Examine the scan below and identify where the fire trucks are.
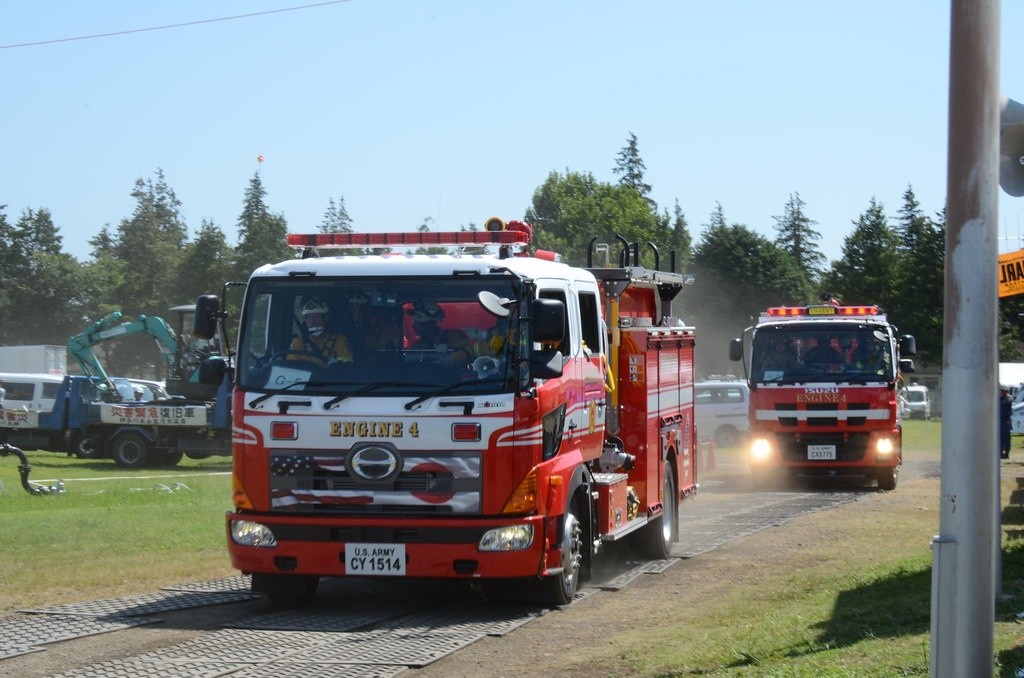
[729,299,916,493]
[193,215,701,615]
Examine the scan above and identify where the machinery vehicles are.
[65,303,233,471]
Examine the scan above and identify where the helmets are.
[345,284,380,304]
[301,299,329,315]
[840,336,851,348]
[407,299,444,323]
[774,336,788,344]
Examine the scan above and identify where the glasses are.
[413,300,424,312]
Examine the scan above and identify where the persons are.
[803,335,840,364]
[999,388,1013,458]
[286,296,354,367]
[762,334,799,371]
[348,285,401,361]
[447,327,503,364]
[855,335,890,374]
[404,300,468,354]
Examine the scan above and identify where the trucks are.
[0,345,68,375]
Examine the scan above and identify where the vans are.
[902,382,930,420]
[0,373,183,451]
[693,373,751,451]
